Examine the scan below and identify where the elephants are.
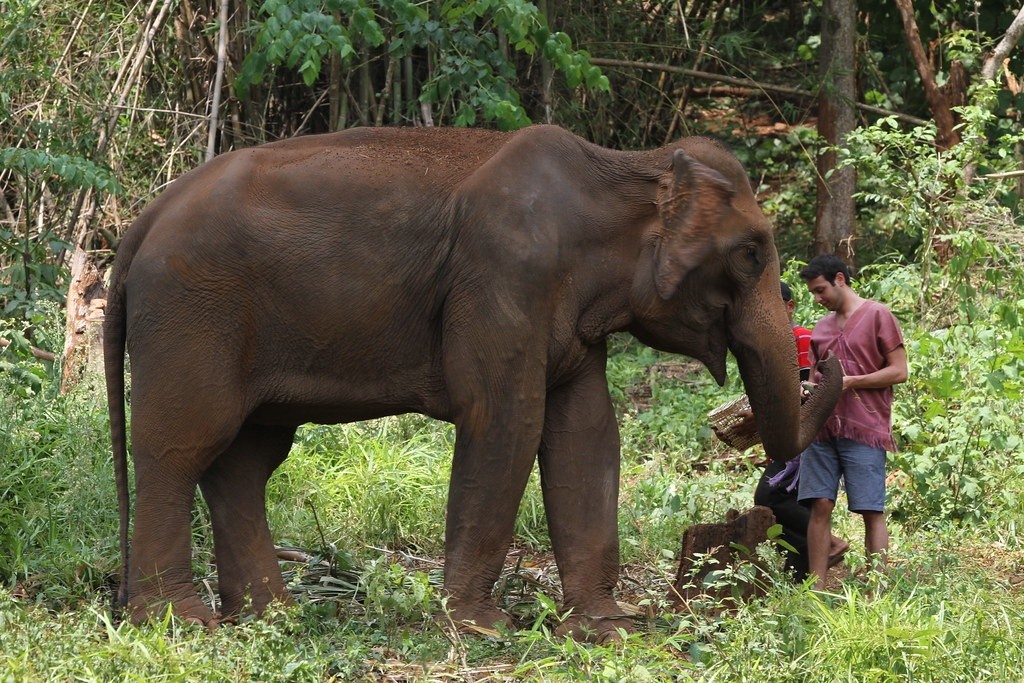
[104,136,844,648]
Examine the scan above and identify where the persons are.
[800,254,908,600]
[733,282,849,584]
